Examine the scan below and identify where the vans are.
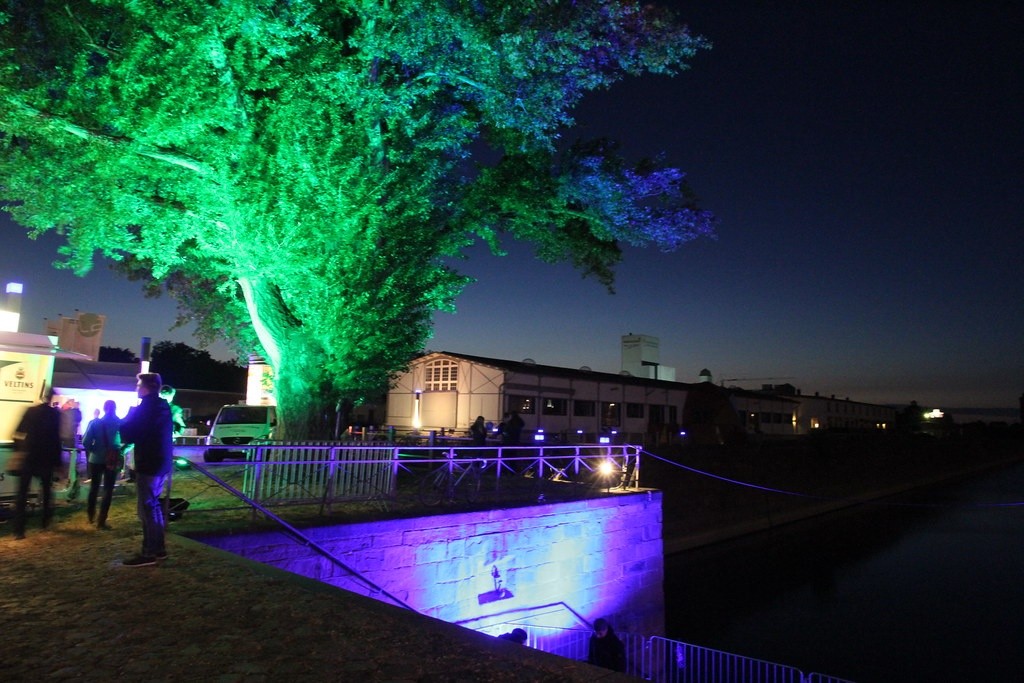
[203,405,279,462]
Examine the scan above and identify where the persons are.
[160,384,185,442]
[52,398,82,448]
[120,373,173,567]
[498,628,527,645]
[13,388,60,541]
[492,411,525,475]
[83,400,120,529]
[84,409,100,459]
[588,618,626,672]
[471,416,488,468]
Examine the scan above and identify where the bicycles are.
[419,452,486,507]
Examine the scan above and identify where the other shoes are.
[155,553,167,560]
[89,519,94,523]
[96,524,112,531]
[15,529,25,539]
[41,518,50,527]
[122,555,155,567]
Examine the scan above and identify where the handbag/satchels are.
[5,452,29,475]
[105,447,124,469]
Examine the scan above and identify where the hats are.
[43,386,58,396]
